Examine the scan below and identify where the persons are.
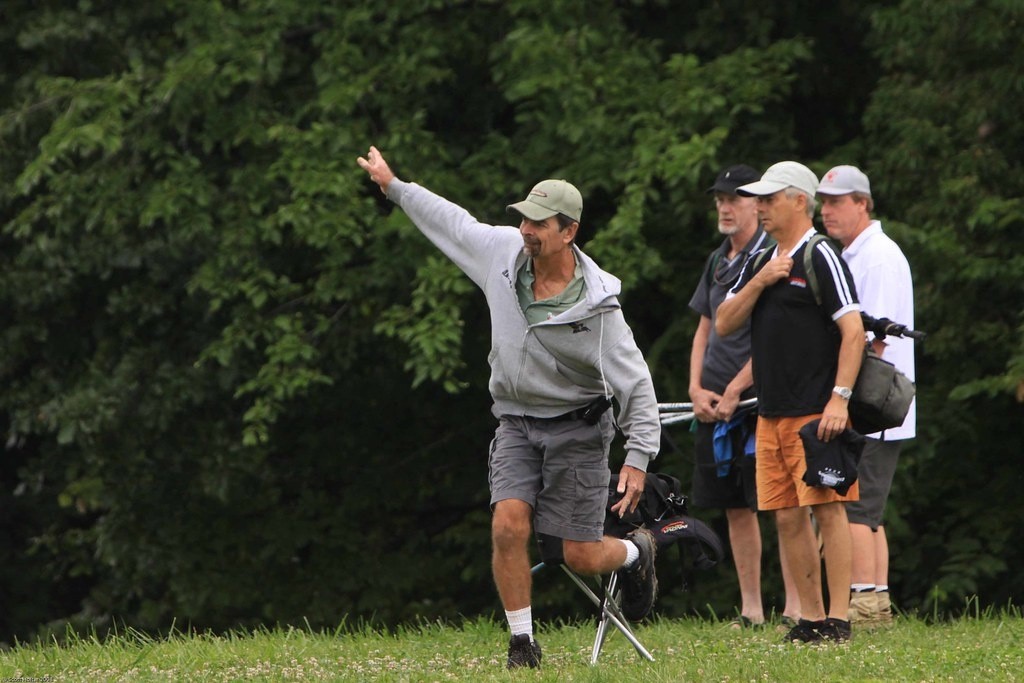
[357,144,661,672]
[814,163,917,631]
[715,160,866,644]
[688,164,802,636]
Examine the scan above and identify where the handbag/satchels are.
[847,352,915,436]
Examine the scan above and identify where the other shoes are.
[848,591,880,627]
[730,616,765,634]
[780,616,797,629]
[878,593,894,627]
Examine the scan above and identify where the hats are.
[736,160,819,197]
[506,180,583,223]
[704,164,762,195]
[817,164,871,195]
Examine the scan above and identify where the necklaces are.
[713,253,748,286]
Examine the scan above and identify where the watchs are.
[832,385,852,399]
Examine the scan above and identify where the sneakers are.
[620,529,658,621]
[812,617,852,647]
[782,618,828,644]
[506,633,542,670]
